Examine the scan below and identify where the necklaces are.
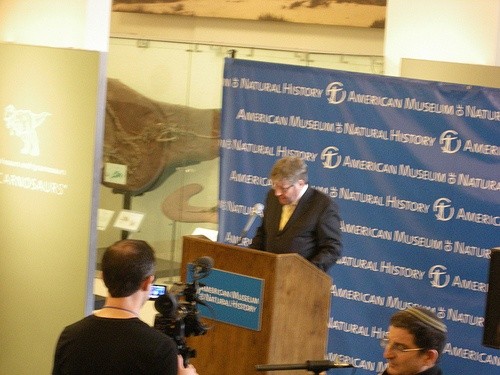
[102,305,139,316]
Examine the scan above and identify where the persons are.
[380,306,448,375]
[246,156,344,271]
[51,239,198,375]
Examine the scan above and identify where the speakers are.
[481,247,500,351]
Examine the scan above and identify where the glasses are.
[276,184,293,192]
[383,332,439,352]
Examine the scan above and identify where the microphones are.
[195,257,213,273]
[238,203,264,245]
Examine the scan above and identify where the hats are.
[404,303,448,333]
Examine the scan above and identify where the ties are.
[278,203,297,232]
